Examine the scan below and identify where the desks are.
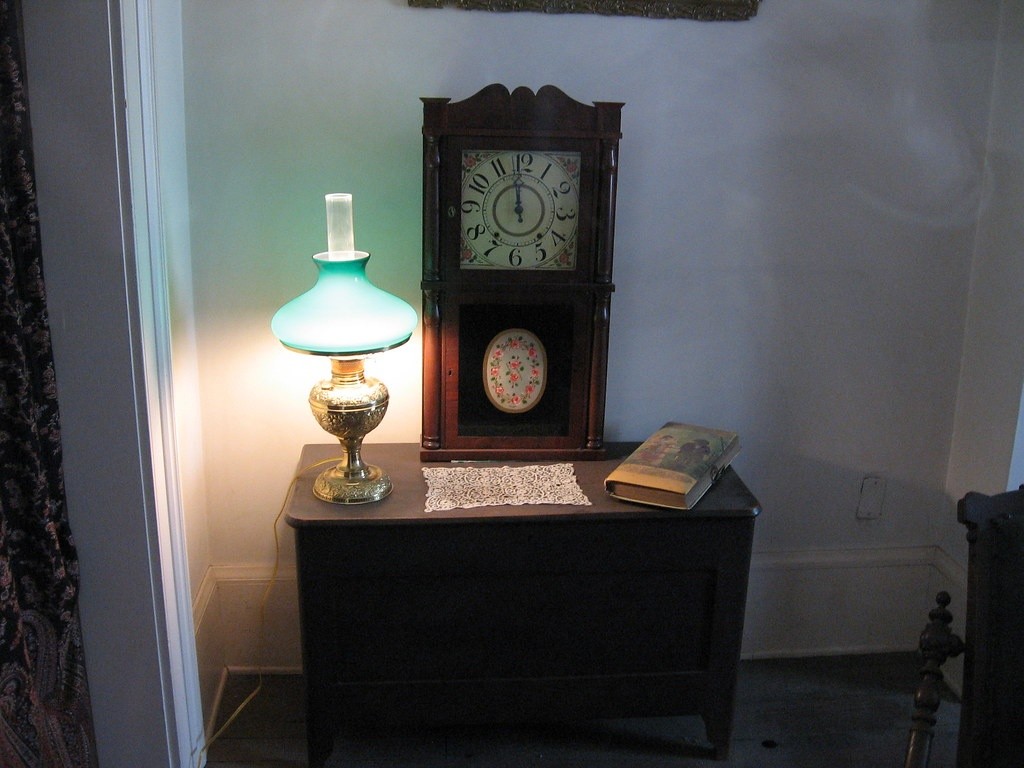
[284,443,760,768]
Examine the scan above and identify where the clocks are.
[419,84,626,462]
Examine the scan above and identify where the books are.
[602,420,743,510]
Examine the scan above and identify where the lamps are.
[272,193,418,505]
